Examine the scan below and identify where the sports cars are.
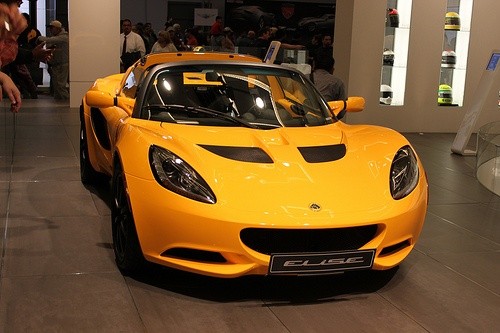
[78,51,430,281]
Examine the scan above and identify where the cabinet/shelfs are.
[438,29,470,108]
[380,23,409,106]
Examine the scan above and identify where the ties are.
[122,36,126,54]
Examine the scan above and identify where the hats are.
[167,27,175,31]
[49,20,62,27]
[224,27,234,32]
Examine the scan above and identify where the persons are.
[0,0,337,113]
[310,52,347,117]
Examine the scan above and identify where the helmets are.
[385,7,399,28]
[445,11,460,30]
[438,84,452,103]
[382,49,394,66]
[441,50,456,68]
[379,84,394,104]
